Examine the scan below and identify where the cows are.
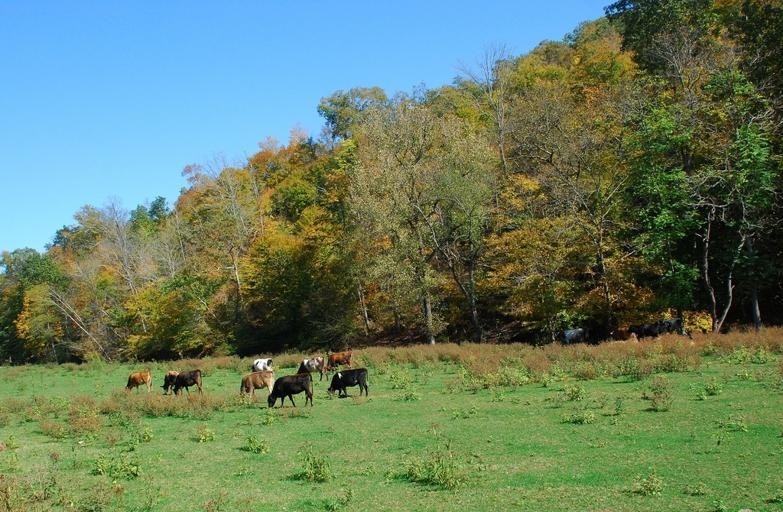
[558,326,593,347]
[160,369,203,398]
[125,371,152,392]
[240,371,273,404]
[627,318,683,338]
[297,357,328,381]
[252,358,274,372]
[326,368,368,399]
[268,373,313,407]
[324,351,352,373]
